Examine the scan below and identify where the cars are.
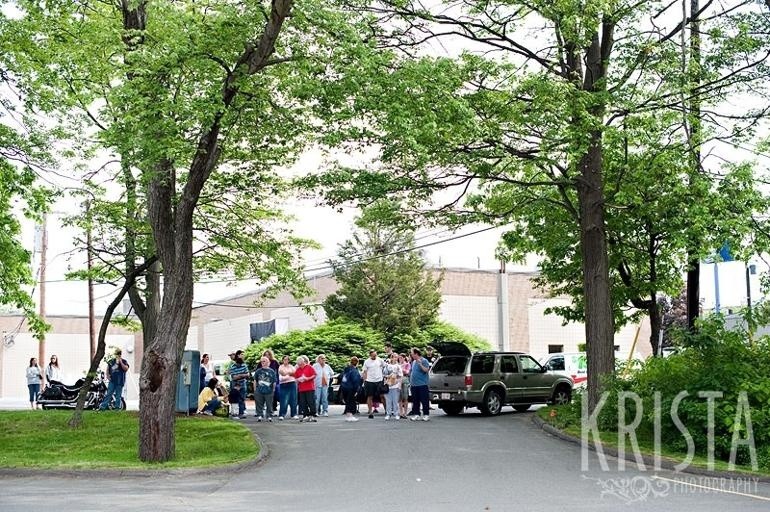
[329,373,367,405]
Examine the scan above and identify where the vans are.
[535,350,647,391]
[207,359,255,401]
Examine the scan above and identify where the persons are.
[46,355,62,388]
[95,348,129,412]
[26,358,42,410]
[196,341,437,422]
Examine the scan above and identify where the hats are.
[228,352,236,356]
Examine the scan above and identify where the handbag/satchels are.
[327,387,333,402]
[214,403,229,418]
[41,385,60,398]
[217,385,229,398]
[257,385,272,394]
[377,381,389,394]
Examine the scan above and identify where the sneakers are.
[315,413,319,417]
[384,411,430,422]
[267,417,273,422]
[368,409,378,418]
[346,417,359,422]
[291,415,317,422]
[203,411,213,416]
[257,416,263,421]
[323,411,328,417]
[231,410,248,420]
[278,415,284,421]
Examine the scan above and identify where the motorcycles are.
[35,367,127,412]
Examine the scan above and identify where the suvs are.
[425,340,574,416]
